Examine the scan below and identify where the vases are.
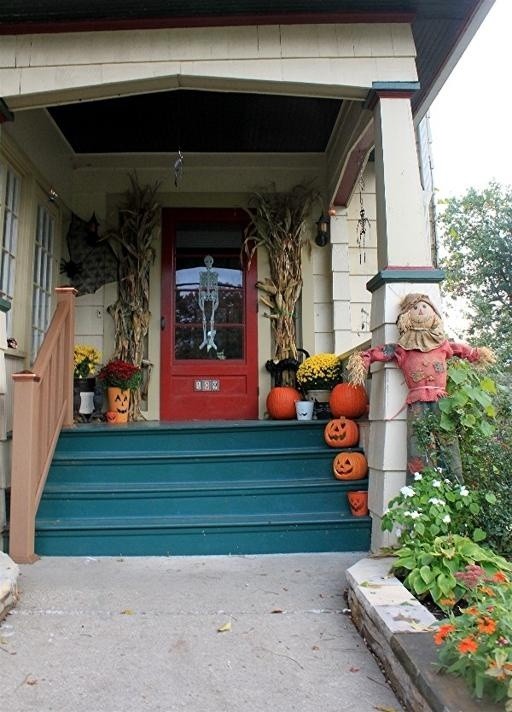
[307,389,335,405]
[74,377,129,424]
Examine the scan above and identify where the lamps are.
[316,208,330,247]
[174,146,185,187]
[86,210,99,245]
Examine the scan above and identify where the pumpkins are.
[329,382,369,418]
[266,386,302,419]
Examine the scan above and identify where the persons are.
[346,292,498,491]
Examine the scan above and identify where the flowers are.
[71,344,136,388]
[297,351,343,391]
